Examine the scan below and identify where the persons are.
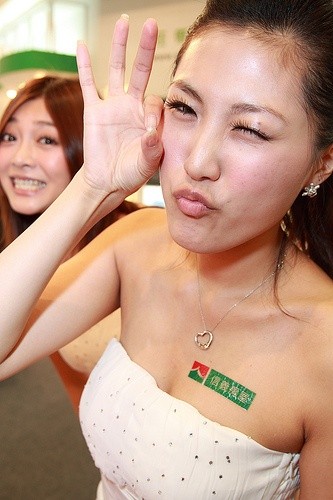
[0,0,333,499]
[0,76,149,417]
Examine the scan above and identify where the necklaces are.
[194,235,290,350]
